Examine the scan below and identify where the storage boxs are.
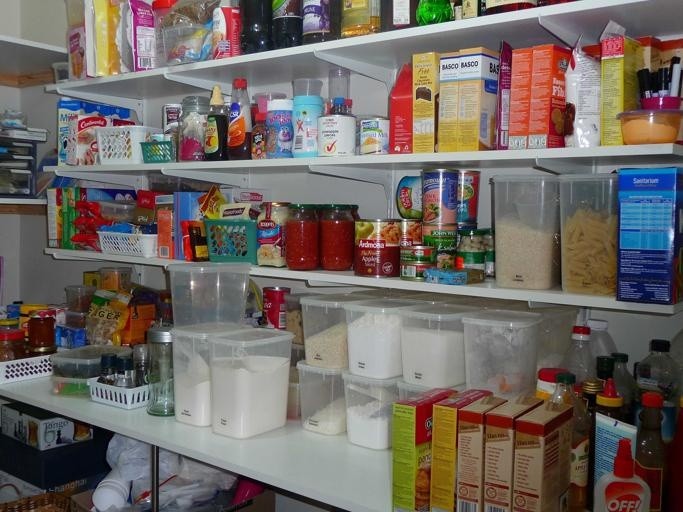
[340,369,401,451]
[401,304,467,389]
[71,431,274,512]
[351,286,426,299]
[459,309,541,396]
[296,360,347,437]
[209,327,295,440]
[343,296,406,380]
[300,291,387,377]
[446,292,530,310]
[163,261,253,335]
[500,298,582,394]
[168,323,254,428]
[616,168,682,303]
[400,290,484,305]
[558,174,618,298]
[489,173,561,291]
[0,401,114,499]
[397,376,435,399]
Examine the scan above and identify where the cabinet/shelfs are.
[0,33,71,504]
[0,0,682,512]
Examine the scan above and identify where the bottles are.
[0,311,57,362]
[177,95,209,159]
[236,0,450,53]
[204,84,228,160]
[101,341,148,388]
[533,320,683,512]
[226,77,254,159]
[263,97,292,158]
[327,95,348,116]
[286,201,362,273]
[292,95,325,156]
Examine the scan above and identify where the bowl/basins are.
[616,109,682,145]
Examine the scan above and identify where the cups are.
[63,284,95,311]
[62,311,88,328]
[253,90,286,113]
[327,65,350,97]
[639,94,682,109]
[146,325,175,417]
[290,77,324,97]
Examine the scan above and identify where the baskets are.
[139,142,172,163]
[85,376,149,409]
[0,482,88,512]
[0,354,51,386]
[205,220,256,263]
[96,231,157,258]
[95,125,163,165]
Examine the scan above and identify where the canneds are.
[255,201,360,272]
[351,166,496,285]
[162,103,183,135]
